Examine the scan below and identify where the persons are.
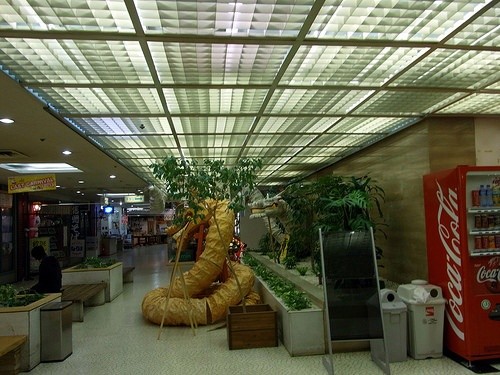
[31,245,62,294]
[156,226,160,243]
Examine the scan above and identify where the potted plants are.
[240,256,325,357]
[147,155,278,350]
[0,283,62,371]
[61,256,124,303]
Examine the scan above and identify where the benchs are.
[60,281,108,321]
[0,335,28,375]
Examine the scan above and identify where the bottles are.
[479,185,486,206]
[485,185,493,206]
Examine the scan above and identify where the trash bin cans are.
[366,288,407,365]
[398,279,446,360]
